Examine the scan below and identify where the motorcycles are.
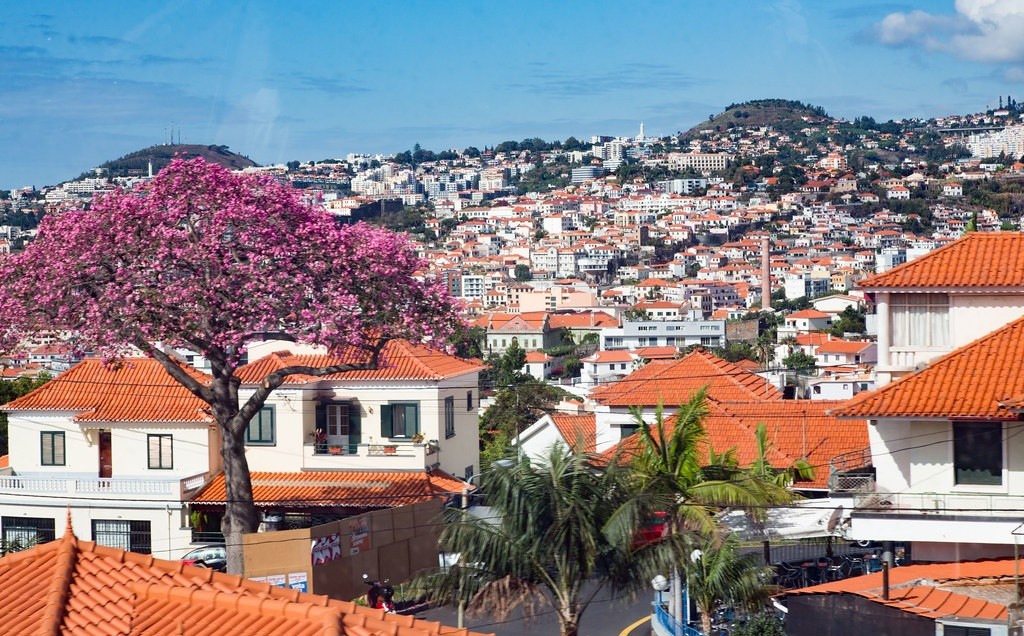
[357,571,398,616]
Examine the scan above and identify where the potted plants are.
[412,432,425,444]
[383,444,397,453]
[328,444,342,454]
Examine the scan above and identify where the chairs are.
[777,554,864,589]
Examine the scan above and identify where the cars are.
[179,543,228,573]
[628,510,674,551]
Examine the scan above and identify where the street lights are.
[449,458,520,629]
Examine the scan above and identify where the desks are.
[863,555,880,575]
[802,563,827,588]
